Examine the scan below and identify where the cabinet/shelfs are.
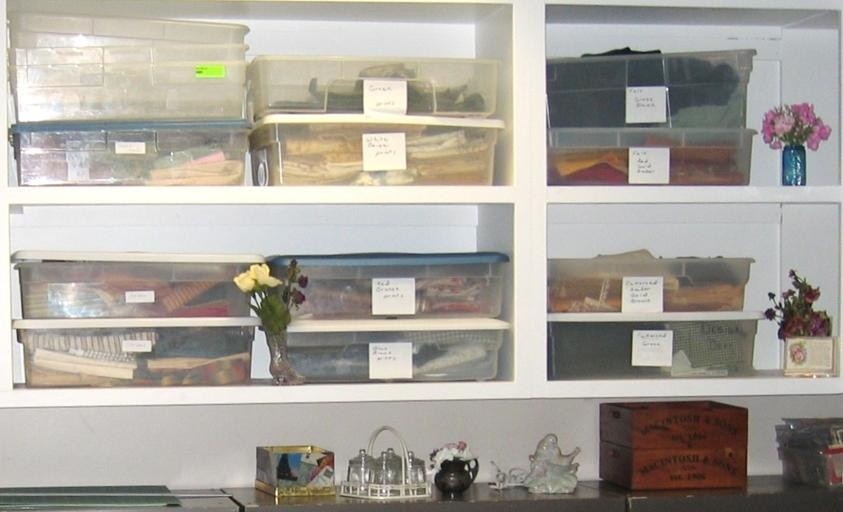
[0,0,843,409]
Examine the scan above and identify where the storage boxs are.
[249,113,506,187]
[7,122,253,186]
[285,318,510,383]
[268,252,510,319]
[599,400,748,491]
[246,54,503,120]
[547,312,766,380]
[8,12,256,123]
[547,258,755,312]
[12,318,265,387]
[547,129,758,185]
[545,50,757,127]
[12,251,266,320]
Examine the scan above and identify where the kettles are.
[348,449,424,493]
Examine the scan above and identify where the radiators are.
[765,269,832,340]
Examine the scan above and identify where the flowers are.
[233,257,309,387]
[761,102,832,151]
[430,441,471,468]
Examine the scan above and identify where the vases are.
[782,146,806,186]
[783,338,835,377]
[435,459,479,500]
